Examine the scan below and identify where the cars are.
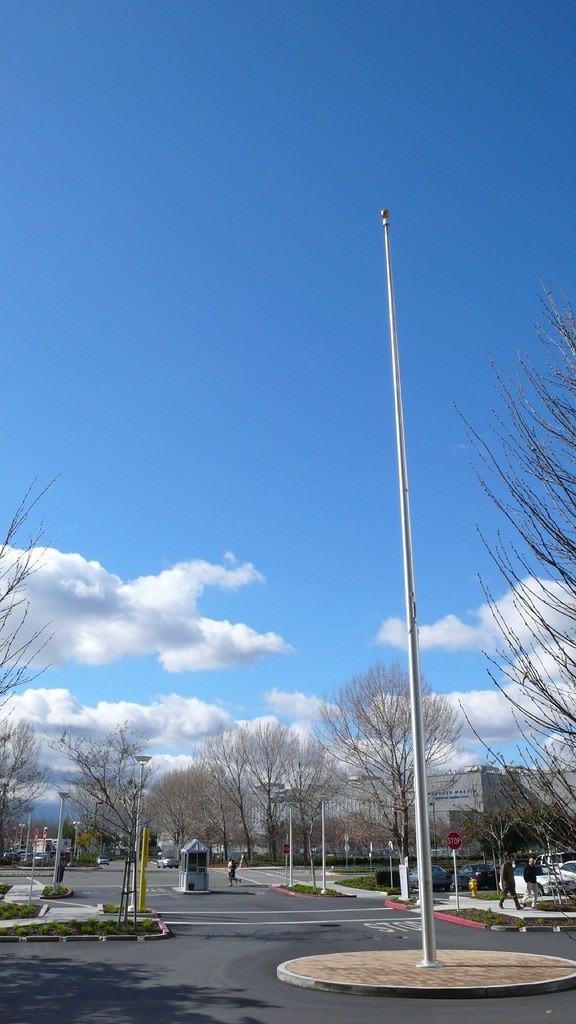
[410,864,450,892]
[491,851,576,897]
[98,855,112,866]
[2,847,71,865]
[450,863,498,889]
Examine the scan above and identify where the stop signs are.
[446,832,463,850]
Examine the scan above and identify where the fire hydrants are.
[468,879,478,898]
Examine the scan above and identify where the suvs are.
[156,856,179,868]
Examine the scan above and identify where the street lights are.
[318,795,332,895]
[19,824,26,851]
[52,791,70,894]
[286,802,296,888]
[126,755,153,913]
[430,801,438,861]
[93,801,104,837]
[72,821,80,854]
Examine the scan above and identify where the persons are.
[225,858,241,887]
[520,856,541,911]
[499,855,525,910]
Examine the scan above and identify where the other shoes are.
[517,906,524,910]
[235,879,238,884]
[521,902,527,907]
[532,907,537,911]
[229,884,233,887]
[498,903,504,909]
[239,878,242,882]
